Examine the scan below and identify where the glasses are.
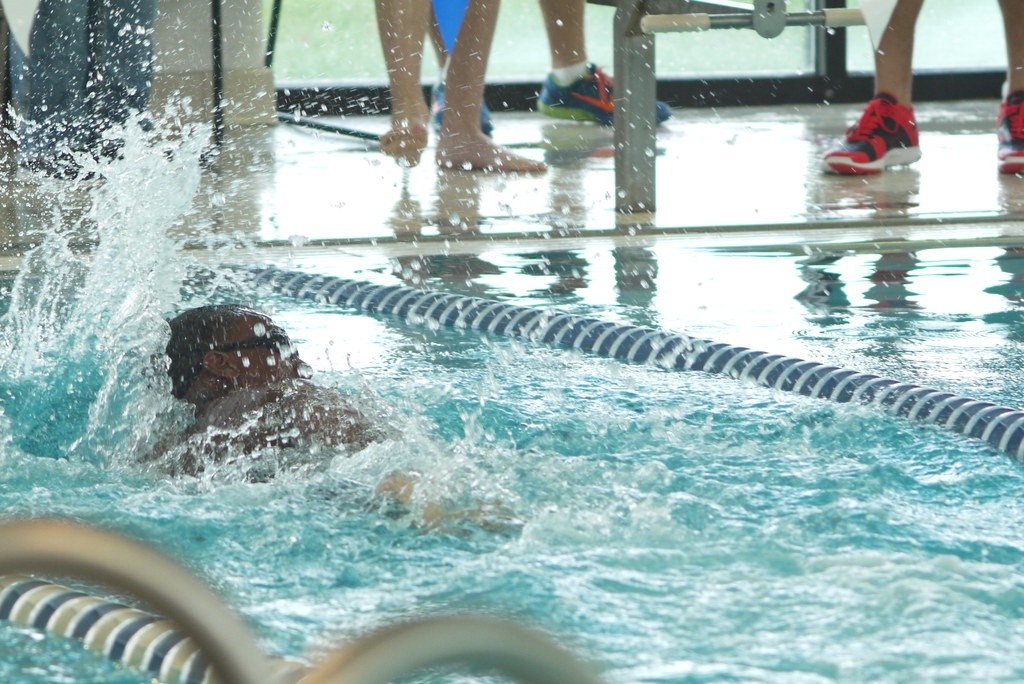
[212,326,298,355]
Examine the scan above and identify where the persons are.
[378,0,672,173]
[130,306,515,530]
[821,0,1024,175]
[9,0,208,180]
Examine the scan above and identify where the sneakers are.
[824,91,922,174]
[536,63,672,126]
[431,74,494,132]
[995,89,1024,173]
